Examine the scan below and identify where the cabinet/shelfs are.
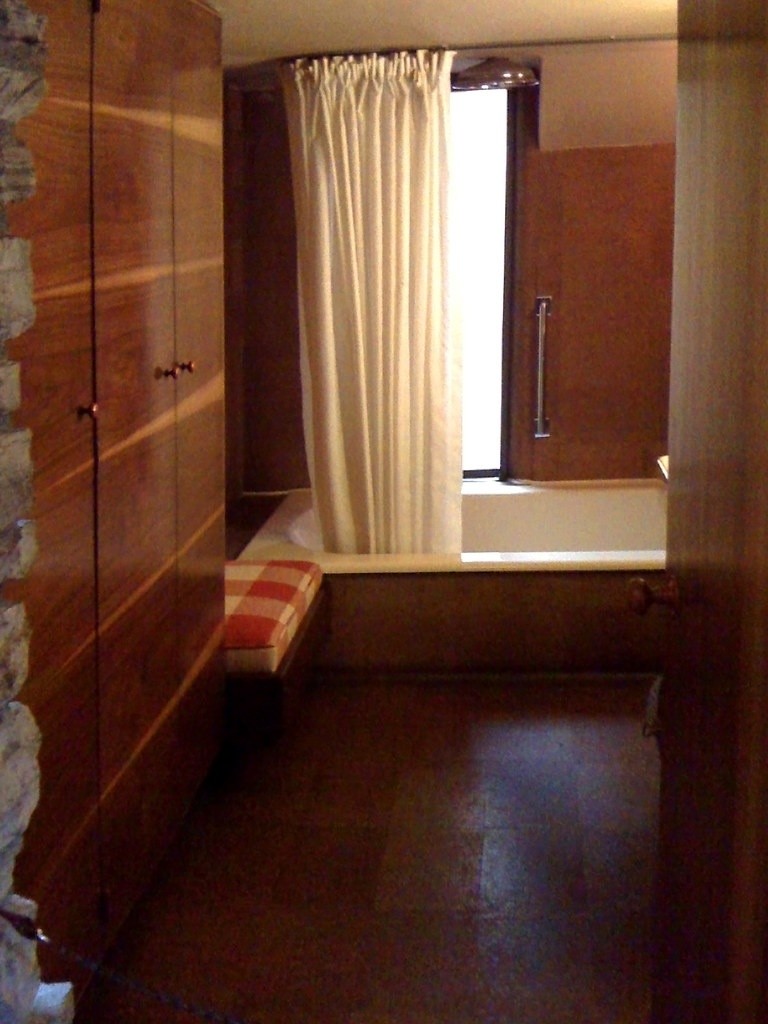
[1,1,220,991]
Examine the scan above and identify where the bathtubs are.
[233,478,668,669]
